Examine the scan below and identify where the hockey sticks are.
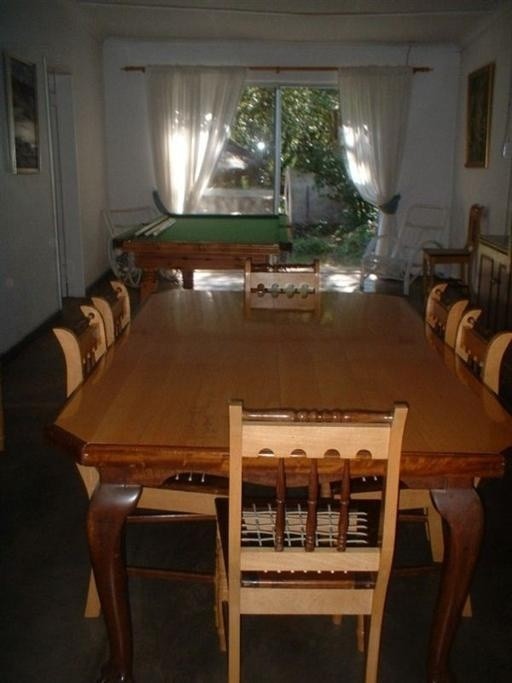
[153,189,279,218]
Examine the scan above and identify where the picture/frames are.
[462,60,496,171]
[2,47,42,178]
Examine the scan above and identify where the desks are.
[111,211,294,289]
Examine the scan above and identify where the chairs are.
[100,205,181,289]
[420,203,486,294]
[359,201,446,297]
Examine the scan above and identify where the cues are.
[134,214,177,237]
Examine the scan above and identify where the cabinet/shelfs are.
[470,232,511,350]
